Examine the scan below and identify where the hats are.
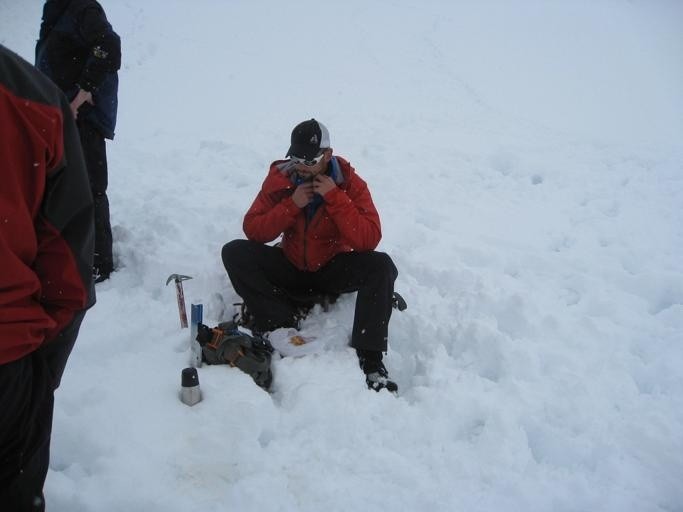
[285,118,332,157]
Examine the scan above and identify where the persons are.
[221,118,398,392]
[34,0,122,285]
[0,43,96,512]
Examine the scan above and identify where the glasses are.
[290,154,324,167]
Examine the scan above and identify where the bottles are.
[181,368,201,405]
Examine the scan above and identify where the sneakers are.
[363,369,399,394]
